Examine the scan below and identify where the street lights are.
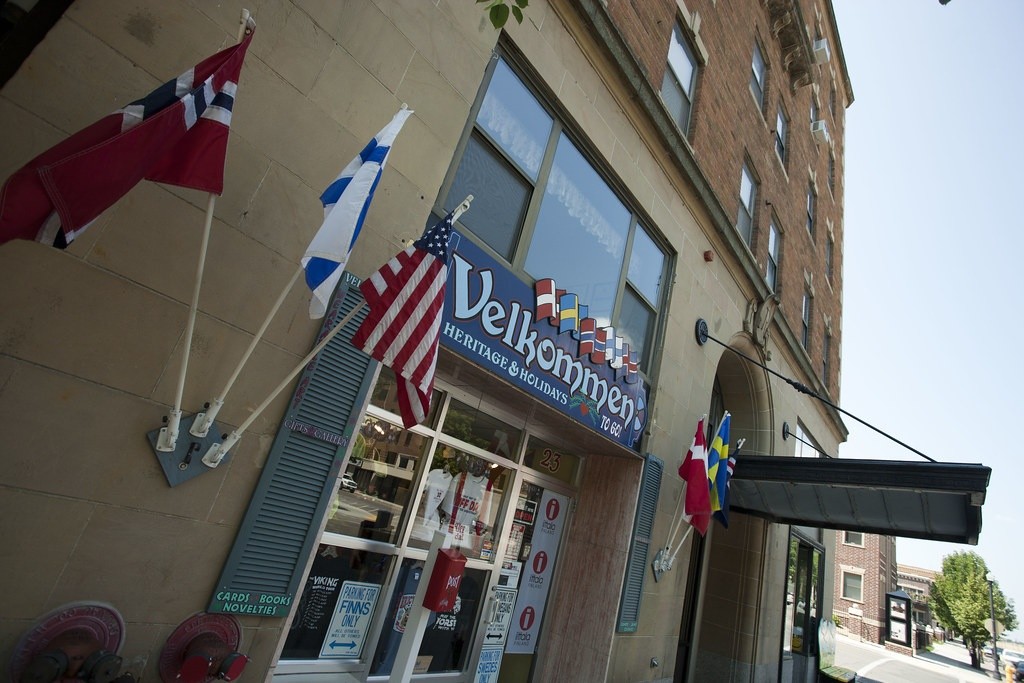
[985,571,1000,680]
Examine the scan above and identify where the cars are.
[984,645,1024,682]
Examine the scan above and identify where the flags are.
[678,413,739,538]
[300,110,415,321]
[0,16,257,249]
[350,209,456,429]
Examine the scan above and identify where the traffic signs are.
[317,581,382,660]
[483,585,518,645]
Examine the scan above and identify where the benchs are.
[818,618,857,683]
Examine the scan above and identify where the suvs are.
[339,474,358,493]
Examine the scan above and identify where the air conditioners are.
[811,120,830,145]
[814,38,831,65]
